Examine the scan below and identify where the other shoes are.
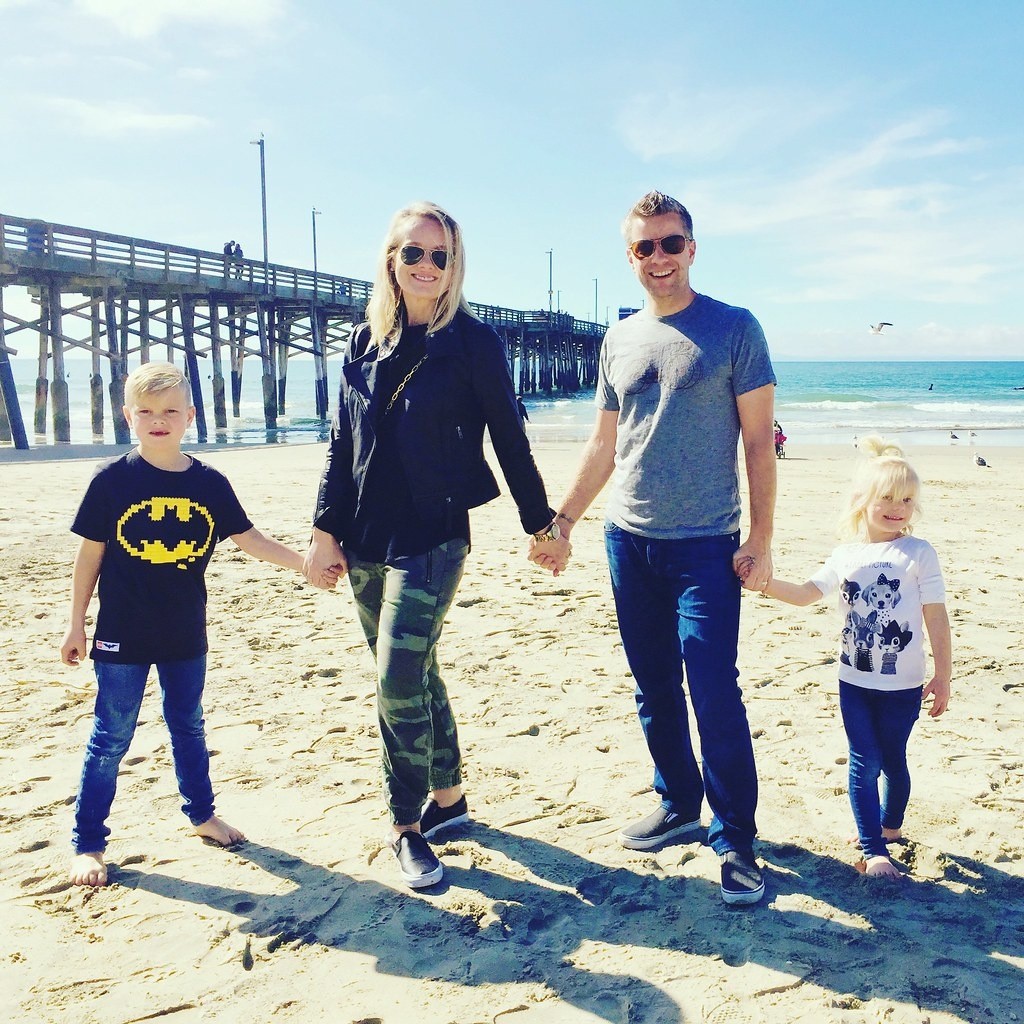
[392,830,443,888]
[420,795,468,838]
[720,848,765,904]
[619,805,702,849]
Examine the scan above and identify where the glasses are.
[398,245,455,271]
[628,235,693,260]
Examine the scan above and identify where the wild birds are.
[972,450,989,466]
[948,430,959,439]
[869,319,891,339]
[969,429,978,437]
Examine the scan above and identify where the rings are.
[762,581,768,586]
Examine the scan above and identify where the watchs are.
[533,522,561,543]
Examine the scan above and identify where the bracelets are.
[556,512,576,524]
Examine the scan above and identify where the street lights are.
[593,277,598,326]
[248,136,271,295]
[546,247,554,314]
[312,208,323,297]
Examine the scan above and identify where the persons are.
[528,188,778,914]
[773,419,787,459]
[58,362,342,885]
[540,309,545,316]
[515,395,530,433]
[489,304,501,319]
[735,435,953,882]
[340,282,347,295]
[301,199,572,889]
[223,240,244,280]
